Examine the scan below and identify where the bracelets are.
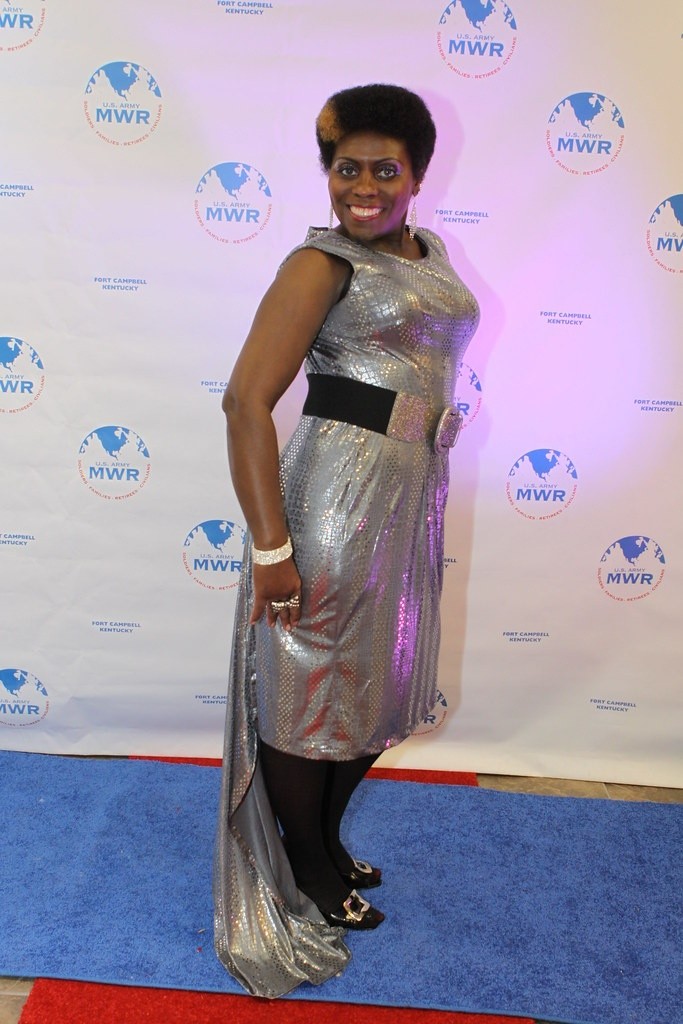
[252,536,293,565]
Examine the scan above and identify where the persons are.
[215,85,480,1001]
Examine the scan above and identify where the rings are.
[271,602,288,613]
[288,595,299,608]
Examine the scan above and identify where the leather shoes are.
[345,851,382,887]
[318,888,385,929]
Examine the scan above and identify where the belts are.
[302,373,463,455]
